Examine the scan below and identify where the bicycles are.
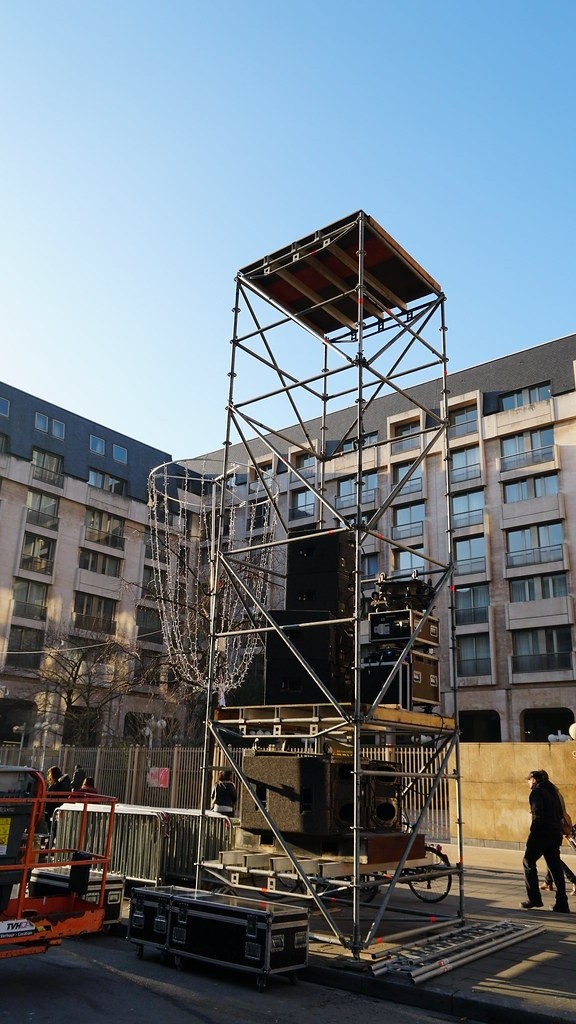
[249,866,329,902]
[350,821,454,906]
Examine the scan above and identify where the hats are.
[525,771,542,781]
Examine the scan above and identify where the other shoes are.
[521,898,544,908]
[570,887,576,897]
[539,882,553,891]
[549,900,570,913]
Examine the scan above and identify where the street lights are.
[147,713,165,785]
[33,722,62,772]
[13,722,27,767]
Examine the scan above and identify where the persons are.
[211,771,238,849]
[519,770,576,912]
[34,765,97,849]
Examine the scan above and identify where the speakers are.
[242,756,404,837]
[262,528,361,705]
